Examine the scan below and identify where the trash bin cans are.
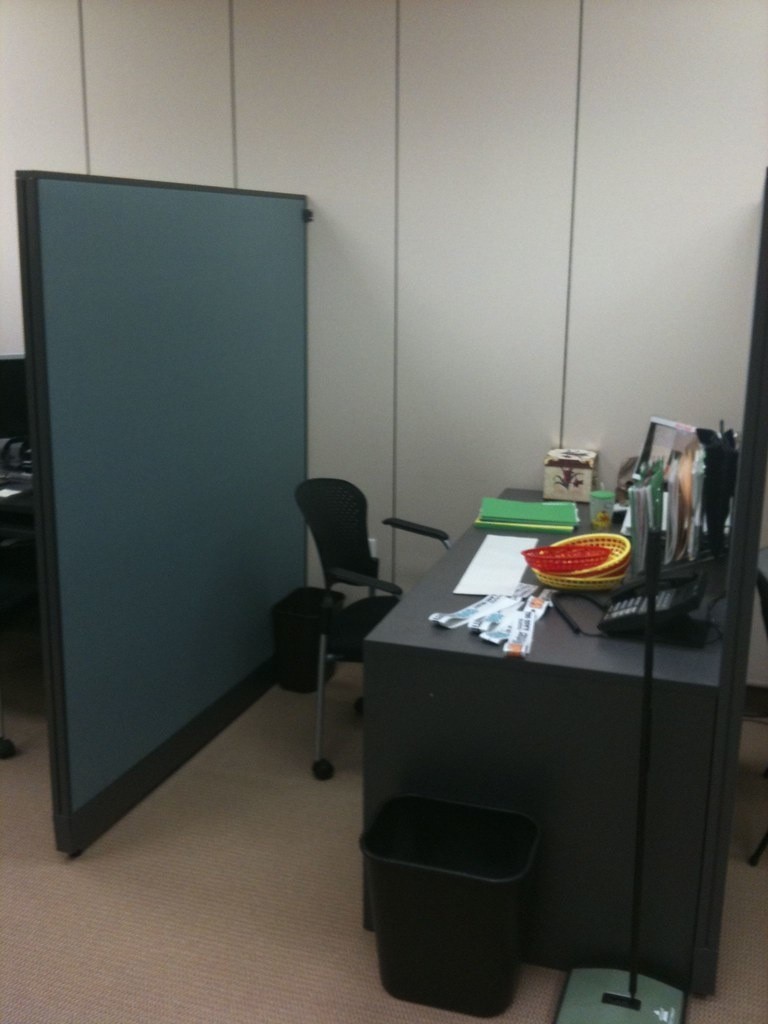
[358,792,537,1017]
[267,587,345,694]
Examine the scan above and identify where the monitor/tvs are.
[632,417,699,482]
[0,355,31,451]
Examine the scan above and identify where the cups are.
[590,491,616,532]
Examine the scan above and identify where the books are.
[626,443,707,581]
[473,495,581,535]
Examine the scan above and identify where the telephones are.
[597,571,705,646]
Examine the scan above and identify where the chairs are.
[270,478,450,782]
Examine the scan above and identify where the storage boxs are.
[543,448,597,503]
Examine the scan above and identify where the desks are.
[359,490,730,998]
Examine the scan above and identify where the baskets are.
[521,532,632,590]
